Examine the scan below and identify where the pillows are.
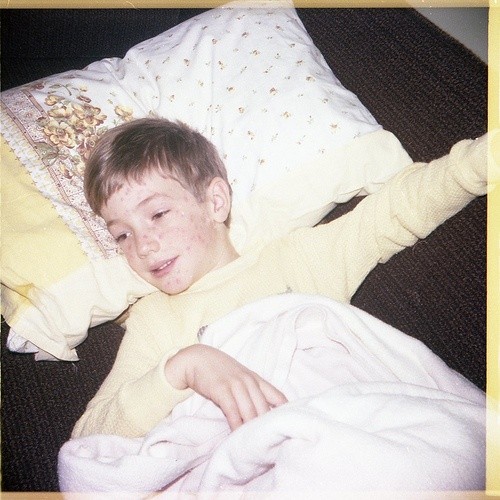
[0,1,413,362]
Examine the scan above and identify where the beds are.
[0,8,486,492]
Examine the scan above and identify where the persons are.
[64,114,487,444]
